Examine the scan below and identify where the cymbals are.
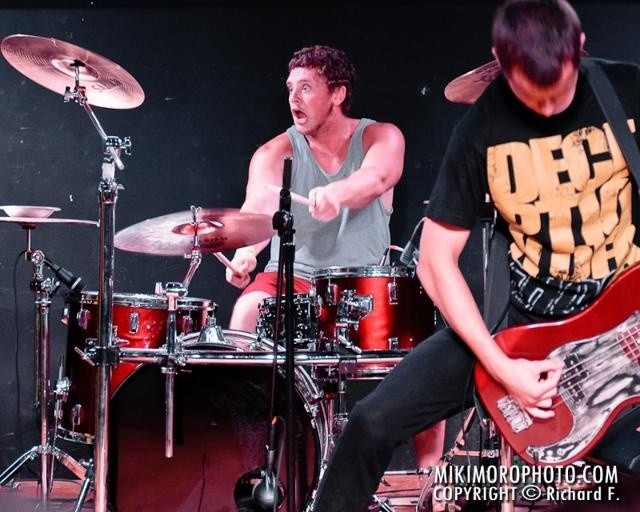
[444,50,589,105]
[114,208,277,256]
[0,205,99,225]
[0,34,146,109]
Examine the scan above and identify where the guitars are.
[474,261,639,470]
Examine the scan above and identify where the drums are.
[308,265,446,386]
[261,295,313,350]
[53,291,221,445]
[97,329,330,512]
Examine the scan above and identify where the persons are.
[225,45,448,498]
[309,2,639,512]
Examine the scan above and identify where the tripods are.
[414,190,565,512]
[0,229,96,511]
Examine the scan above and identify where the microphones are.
[44,257,86,293]
[253,412,285,508]
[399,221,423,265]
